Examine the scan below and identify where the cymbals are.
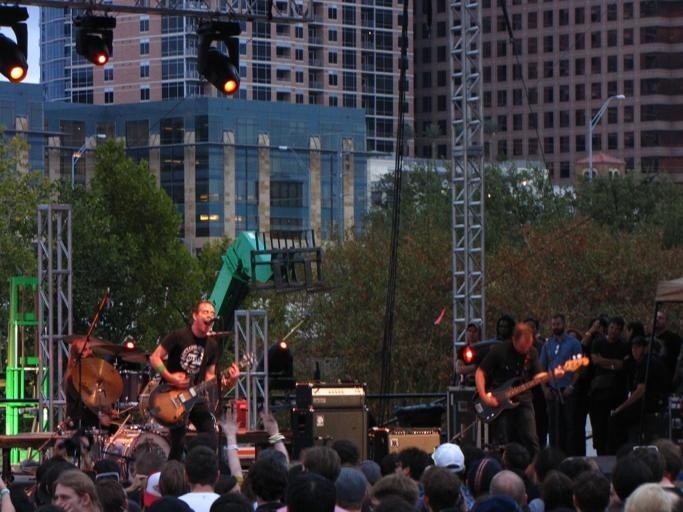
[72,358,124,409]
[88,342,147,362]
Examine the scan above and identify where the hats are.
[467,458,501,497]
[432,443,464,474]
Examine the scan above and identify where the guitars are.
[148,352,257,426]
[471,354,589,422]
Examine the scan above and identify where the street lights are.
[70,132,107,193]
[584,94,626,182]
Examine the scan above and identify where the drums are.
[116,369,150,409]
[103,428,171,486]
[139,379,169,433]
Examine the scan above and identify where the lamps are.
[0,2,240,95]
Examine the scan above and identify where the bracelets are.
[154,364,165,373]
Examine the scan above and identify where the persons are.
[454,308,682,463]
[61,337,120,458]
[148,300,240,461]
[1,407,683,512]
